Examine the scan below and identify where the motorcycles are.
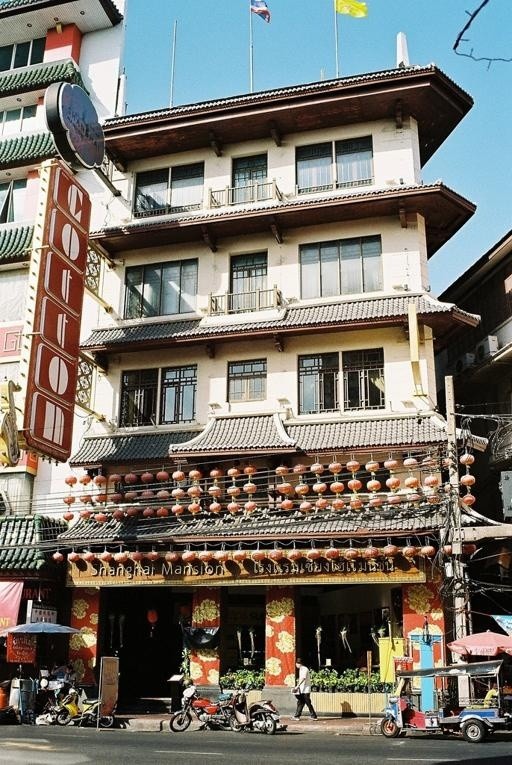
[169,679,280,736]
[380,660,512,743]
[53,686,117,728]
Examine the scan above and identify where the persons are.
[52,659,79,706]
[290,657,318,721]
[484,684,499,709]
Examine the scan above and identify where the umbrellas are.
[447,630,512,691]
[0,617,86,635]
